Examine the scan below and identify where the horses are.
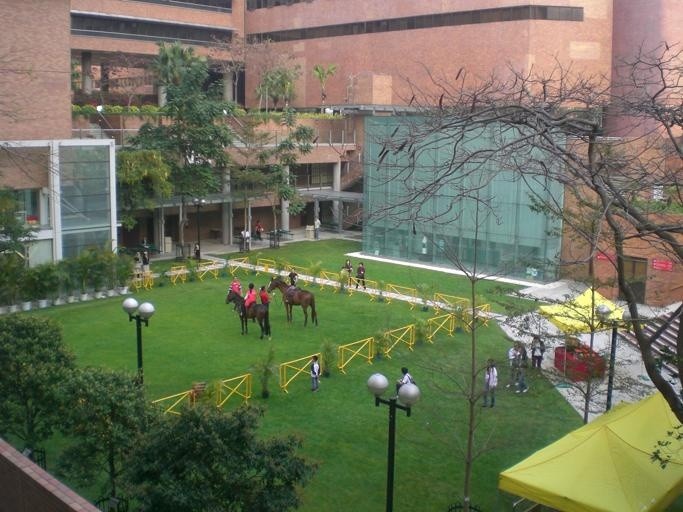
[225,290,272,341]
[267,275,317,326]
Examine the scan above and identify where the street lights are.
[596,305,634,411]
[192,197,207,248]
[366,372,421,511]
[123,297,155,384]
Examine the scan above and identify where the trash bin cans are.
[164,236,172,253]
[305,225,315,239]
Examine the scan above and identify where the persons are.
[345,259,353,287]
[194,243,200,261]
[289,268,298,286]
[356,263,366,289]
[256,220,262,239]
[314,216,320,240]
[506,337,545,394]
[133,251,150,277]
[259,286,271,306]
[310,353,321,390]
[240,283,256,314]
[230,276,242,312]
[482,359,498,408]
[396,367,413,397]
